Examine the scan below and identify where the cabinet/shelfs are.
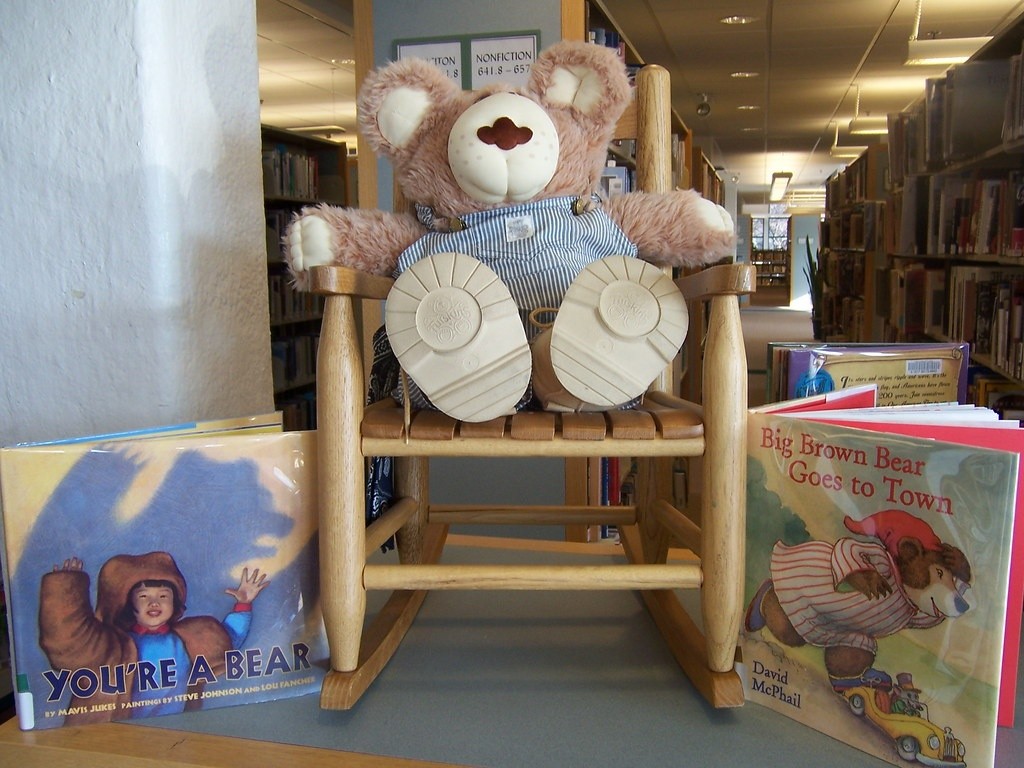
[809,12,1023,423]
[738,213,753,309]
[259,120,351,429]
[785,215,821,312]
[355,2,726,550]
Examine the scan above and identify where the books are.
[731,50,1024,767]
[0,409,341,731]
[262,138,321,433]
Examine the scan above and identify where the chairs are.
[305,63,753,715]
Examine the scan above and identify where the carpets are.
[115,312,1022,768]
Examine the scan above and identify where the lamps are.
[828,123,867,161]
[694,90,713,116]
[769,154,793,203]
[899,0,994,64]
[847,87,888,136]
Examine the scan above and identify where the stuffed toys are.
[278,41,745,447]
